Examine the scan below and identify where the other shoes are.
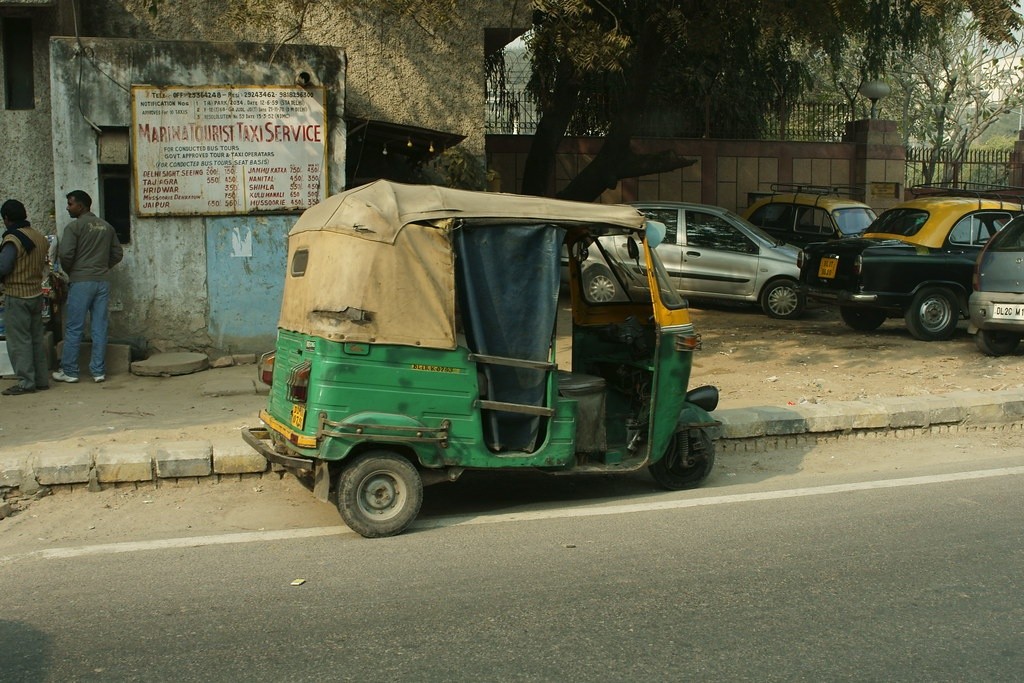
[1,385,35,395]
[37,384,50,390]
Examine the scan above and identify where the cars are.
[962,209,1024,358]
[738,182,885,249]
[794,181,1024,343]
[562,200,806,322]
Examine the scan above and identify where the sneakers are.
[52,369,79,382]
[93,373,106,382]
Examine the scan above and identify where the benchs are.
[557,369,607,464]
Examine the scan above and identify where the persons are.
[53,190,124,383]
[0,197,52,396]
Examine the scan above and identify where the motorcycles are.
[238,176,725,540]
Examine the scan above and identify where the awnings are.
[346,116,465,164]
